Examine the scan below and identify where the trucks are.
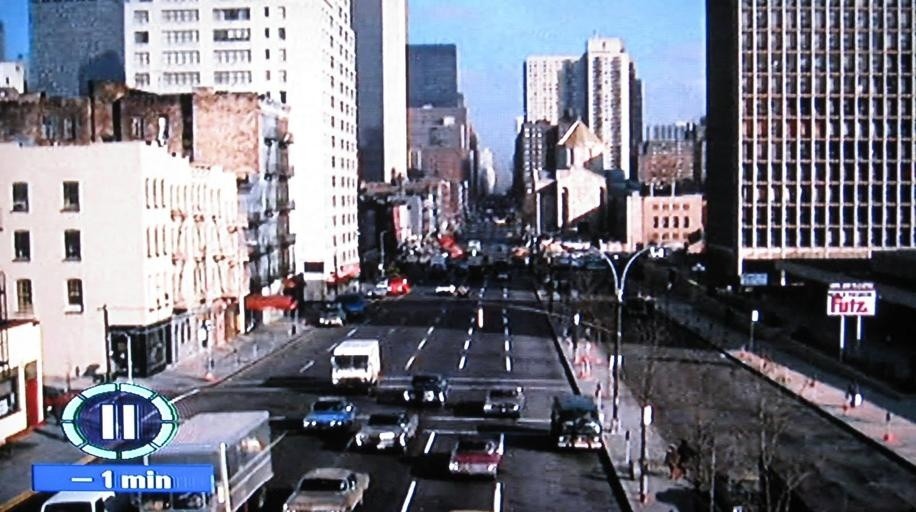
[329,338,381,394]
[129,409,274,512]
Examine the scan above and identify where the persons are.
[847,378,863,409]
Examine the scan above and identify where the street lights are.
[334,229,361,300]
[691,263,706,323]
[561,240,685,436]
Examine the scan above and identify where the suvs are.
[550,394,602,452]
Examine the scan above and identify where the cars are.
[319,303,346,327]
[332,293,373,320]
[42,384,83,411]
[403,372,452,408]
[483,384,526,419]
[282,467,370,512]
[303,394,357,433]
[448,431,505,478]
[355,408,419,452]
[40,490,116,511]
